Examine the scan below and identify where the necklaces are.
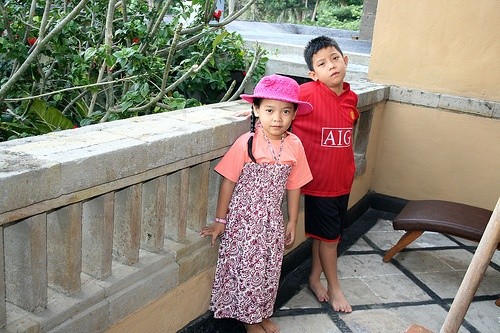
[260,123,285,166]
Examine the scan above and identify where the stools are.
[382,200,500,307]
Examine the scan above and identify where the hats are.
[238,74,314,114]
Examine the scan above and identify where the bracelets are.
[214,217,227,225]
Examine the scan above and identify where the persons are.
[200,74,313,333]
[288,34,359,313]
